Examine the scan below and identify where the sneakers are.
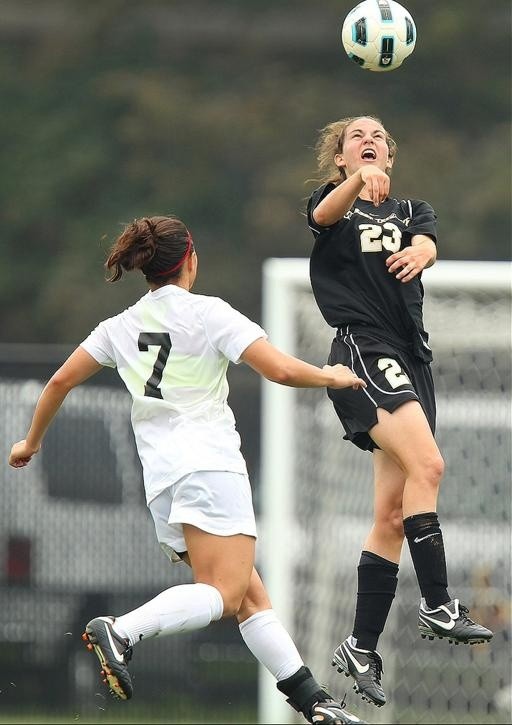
[331,634,386,708]
[81,615,133,702]
[310,699,367,724]
[417,597,493,645]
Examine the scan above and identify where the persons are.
[9,216,367,725]
[306,116,493,706]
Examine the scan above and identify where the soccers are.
[342,0,419,75]
